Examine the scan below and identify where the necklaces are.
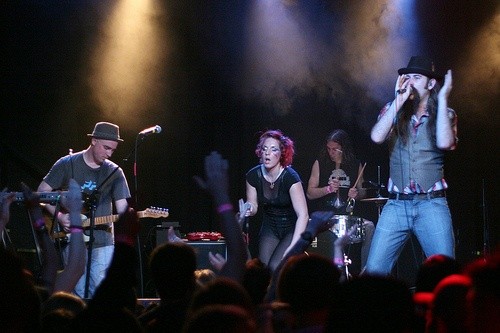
[267,163,283,189]
[416,108,426,119]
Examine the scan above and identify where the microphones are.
[397,87,415,95]
[244,202,250,227]
[134,125,162,136]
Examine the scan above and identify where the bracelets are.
[37,227,47,234]
[34,218,44,227]
[70,224,84,228]
[72,229,83,232]
[328,186,331,193]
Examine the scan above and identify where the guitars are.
[44,205,169,239]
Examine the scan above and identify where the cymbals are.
[360,196,389,202]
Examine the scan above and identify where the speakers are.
[186,241,228,274]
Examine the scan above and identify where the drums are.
[328,214,366,244]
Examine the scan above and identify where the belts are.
[390,189,446,199]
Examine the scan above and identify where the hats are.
[397,55,439,79]
[86,122,124,143]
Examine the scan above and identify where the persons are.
[36,123,134,303]
[0,151,500,333]
[244,131,309,298]
[307,130,374,273]
[359,56,458,276]
